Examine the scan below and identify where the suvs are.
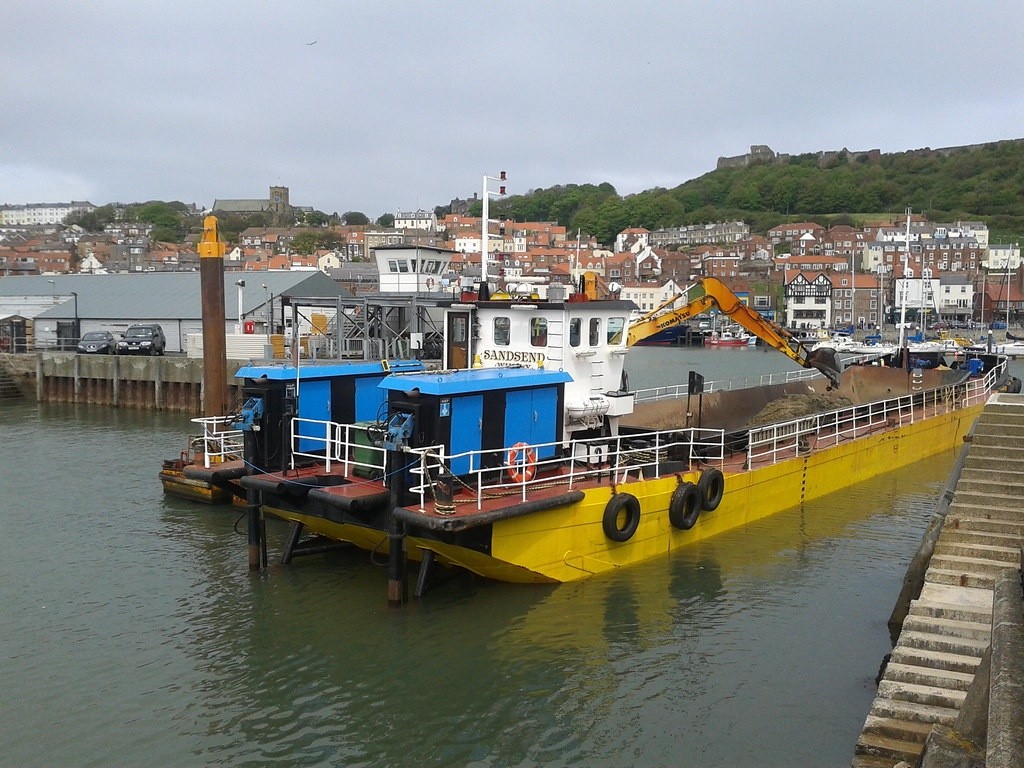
[116,325,167,355]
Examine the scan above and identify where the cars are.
[76,330,116,355]
[927,323,950,330]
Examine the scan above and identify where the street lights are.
[263,284,269,333]
[48,280,56,302]
[235,279,246,333]
[479,169,506,300]
[70,291,80,338]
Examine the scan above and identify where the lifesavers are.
[602,492,641,542]
[697,468,725,512]
[507,441,536,483]
[668,481,702,530]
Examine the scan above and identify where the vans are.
[970,322,986,330]
[949,320,969,329]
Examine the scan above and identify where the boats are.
[228,332,1008,593]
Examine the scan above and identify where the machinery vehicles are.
[573,271,844,395]
